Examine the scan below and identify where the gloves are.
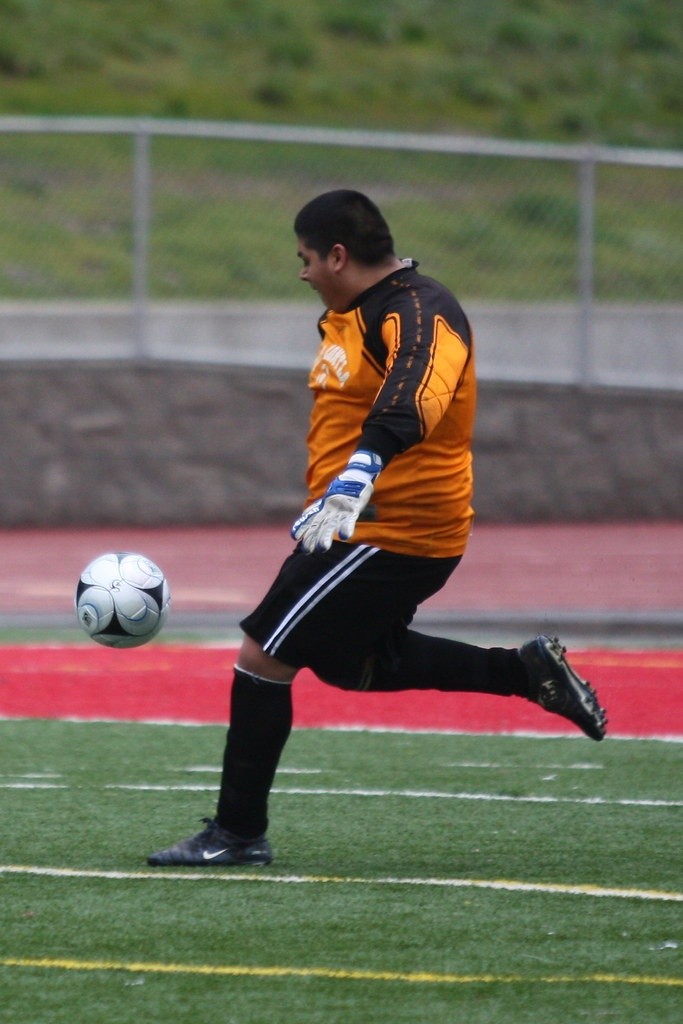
[291,450,382,556]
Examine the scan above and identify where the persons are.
[145,191,607,869]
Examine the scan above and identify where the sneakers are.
[517,635,608,742]
[147,817,271,867]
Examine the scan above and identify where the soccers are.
[74,551,171,651]
